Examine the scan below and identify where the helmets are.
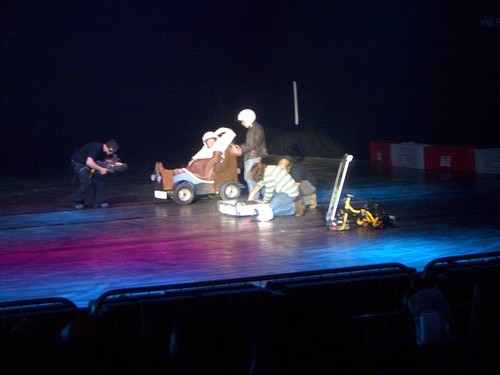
[238,109,256,128]
[202,131,218,148]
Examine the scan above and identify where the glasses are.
[109,147,113,153]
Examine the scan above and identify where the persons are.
[228,108,317,217]
[72,140,128,209]
[155,130,222,181]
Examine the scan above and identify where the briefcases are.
[218,198,264,217]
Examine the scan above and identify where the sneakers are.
[295,200,305,216]
[310,194,317,209]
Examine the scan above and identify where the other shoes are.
[100,202,109,208]
[75,204,83,209]
